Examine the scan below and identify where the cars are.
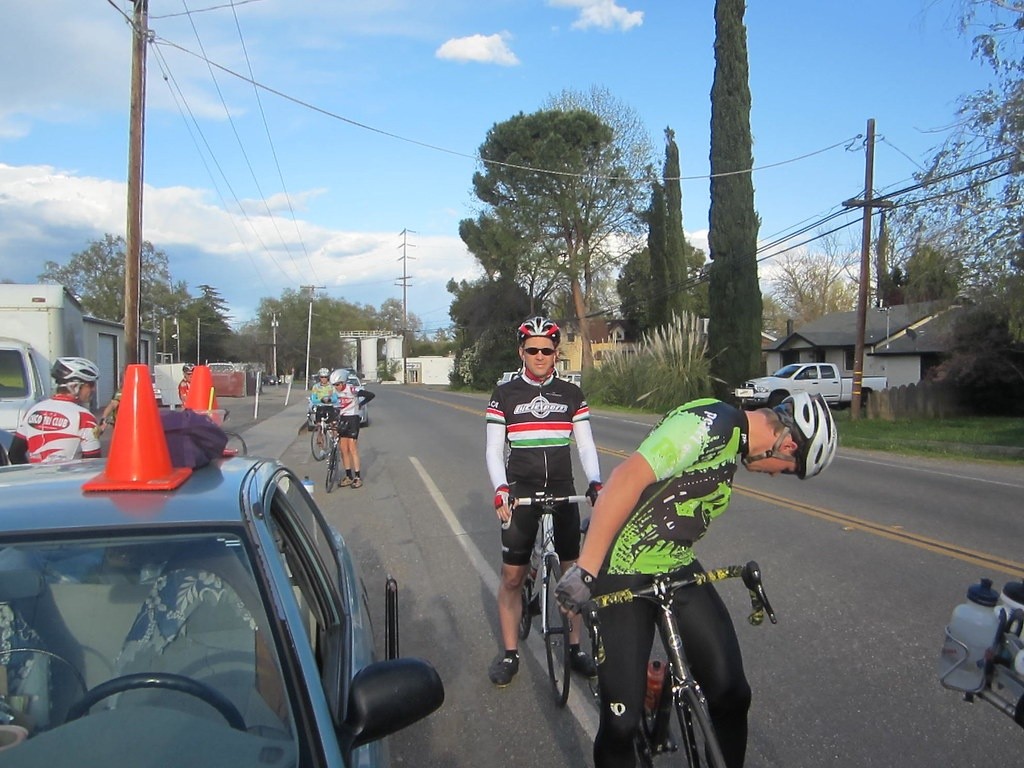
[262,374,282,386]
[307,376,368,432]
[0,454,446,768]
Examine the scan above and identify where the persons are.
[554,390,837,768]
[6,356,104,464]
[99,384,123,426]
[483,318,606,688]
[327,369,376,488]
[311,367,337,459]
[177,363,195,407]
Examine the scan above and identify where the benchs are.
[26,580,146,713]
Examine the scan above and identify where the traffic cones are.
[80,363,194,493]
[182,365,220,426]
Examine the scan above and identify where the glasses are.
[84,381,95,389]
[320,376,326,378]
[524,347,556,356]
[333,384,341,387]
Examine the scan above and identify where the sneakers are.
[340,476,354,486]
[351,477,362,488]
[491,653,519,687]
[570,651,597,677]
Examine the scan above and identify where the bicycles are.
[306,395,348,494]
[581,559,776,768]
[219,408,248,457]
[502,487,572,710]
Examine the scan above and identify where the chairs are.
[119,569,261,732]
[0,599,55,739]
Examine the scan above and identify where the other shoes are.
[319,449,326,457]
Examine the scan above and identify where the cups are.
[194,410,226,430]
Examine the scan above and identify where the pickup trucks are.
[734,362,888,410]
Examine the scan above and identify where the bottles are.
[300,476,315,496]
[530,545,541,579]
[644,659,665,715]
[937,577,999,692]
[992,577,1024,640]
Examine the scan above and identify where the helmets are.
[50,357,101,382]
[329,369,349,385]
[318,368,330,377]
[183,363,195,373]
[517,316,561,349]
[783,391,838,480]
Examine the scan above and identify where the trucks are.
[0,283,85,434]
